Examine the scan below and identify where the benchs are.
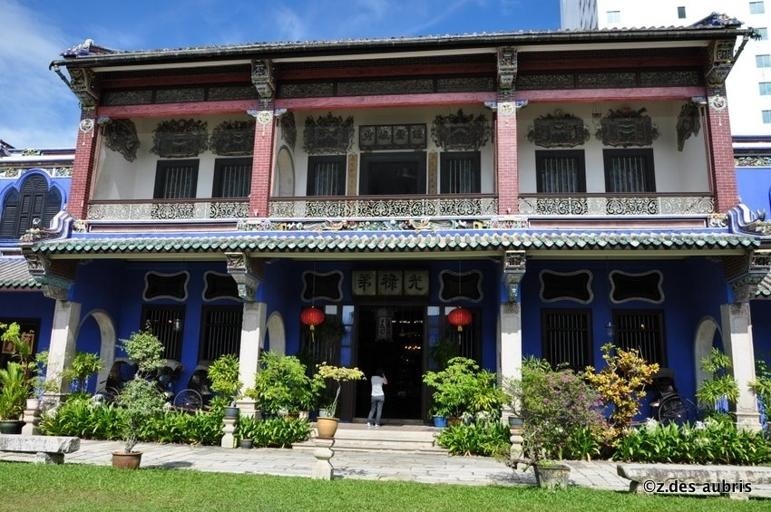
[1,433,83,473]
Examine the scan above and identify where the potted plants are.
[1,321,39,433]
[421,355,528,426]
[111,331,165,472]
[220,347,368,450]
[533,457,572,490]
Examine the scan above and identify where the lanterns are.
[300,305,325,330]
[448,306,472,332]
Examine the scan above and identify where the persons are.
[367,368,388,427]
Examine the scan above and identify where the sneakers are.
[367,422,380,428]
